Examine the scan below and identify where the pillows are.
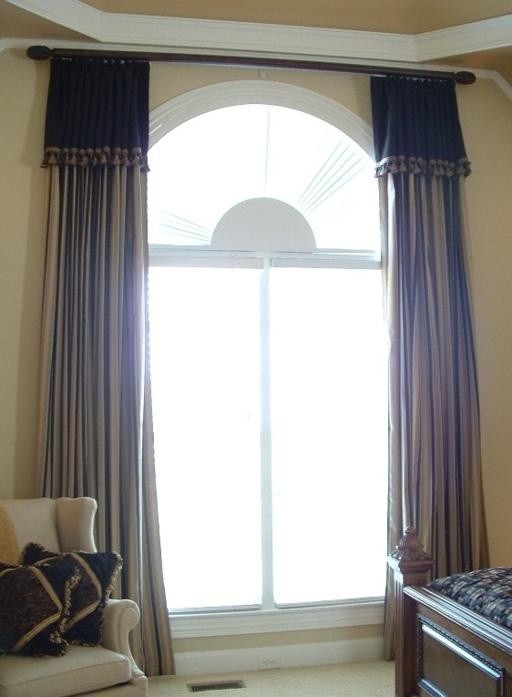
[0,552,82,659]
[20,542,124,647]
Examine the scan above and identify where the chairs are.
[0,497,149,697]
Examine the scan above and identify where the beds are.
[387,525,512,697]
